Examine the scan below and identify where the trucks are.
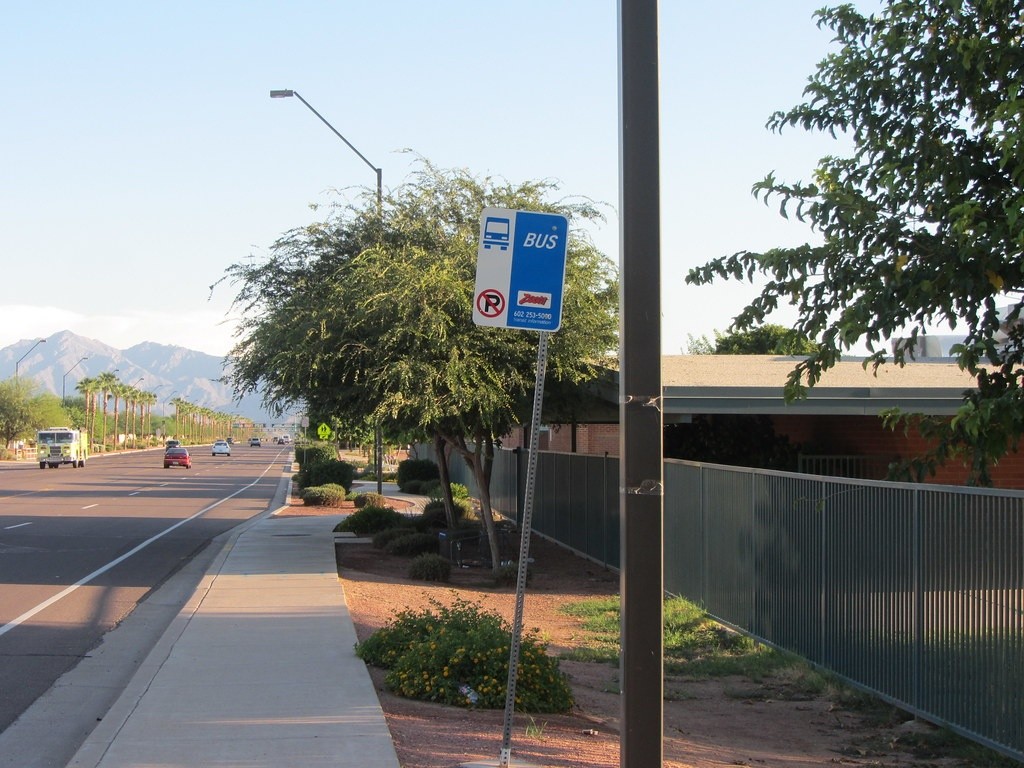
[37,428,88,468]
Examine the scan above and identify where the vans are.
[282,435,291,443]
[166,440,180,451]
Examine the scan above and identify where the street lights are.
[269,90,382,496]
[63,358,89,406]
[16,339,47,383]
[163,391,178,446]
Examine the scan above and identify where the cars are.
[278,439,284,445]
[163,448,192,469]
[226,438,234,445]
[212,440,230,456]
[273,437,278,442]
[250,438,261,447]
